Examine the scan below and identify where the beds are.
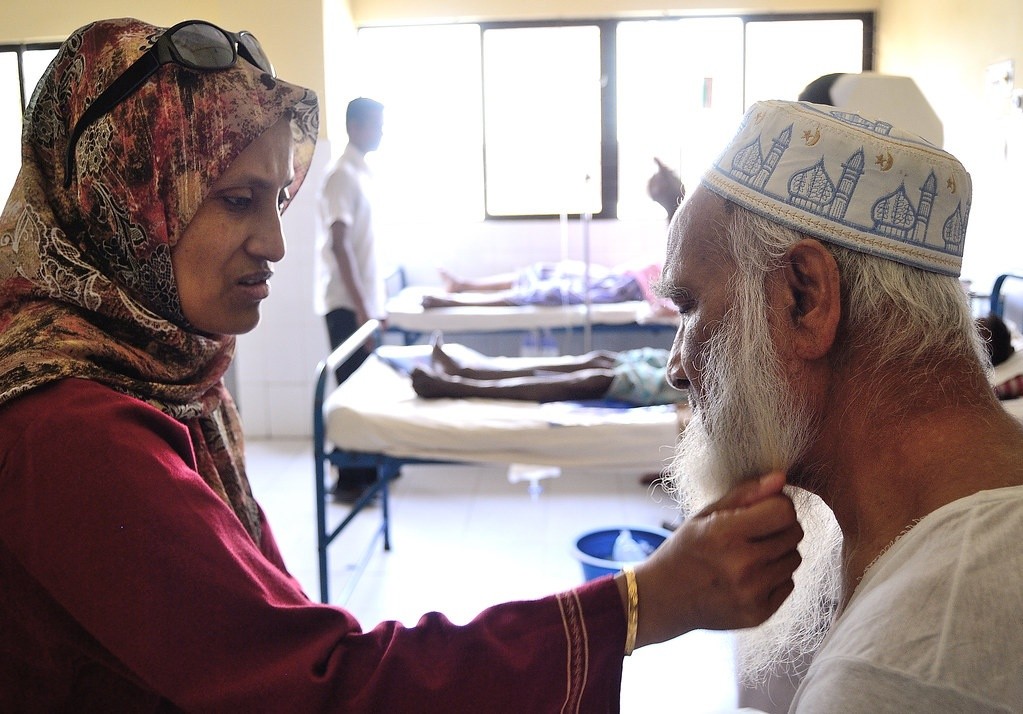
[313,262,1023,608]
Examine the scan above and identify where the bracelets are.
[622,567,638,656]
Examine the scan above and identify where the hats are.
[798,73,943,149]
[702,99,974,278]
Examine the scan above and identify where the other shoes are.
[333,467,402,504]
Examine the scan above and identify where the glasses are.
[63,20,276,191]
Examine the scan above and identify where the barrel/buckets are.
[571,525,669,581]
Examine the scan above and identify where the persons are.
[410,314,1014,403]
[0,18,803,714]
[646,71,943,218]
[313,99,389,489]
[654,100,1023,714]
[420,264,662,309]
